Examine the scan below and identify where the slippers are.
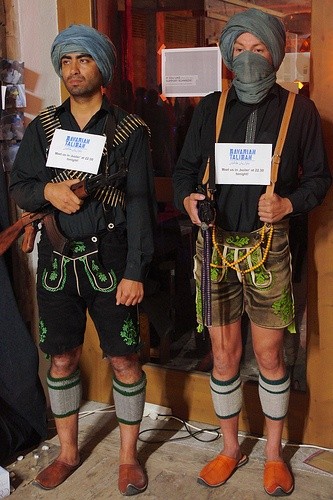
[197,453,249,487]
[263,456,295,496]
[119,456,148,495]
[32,452,87,490]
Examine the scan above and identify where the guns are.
[0,167,128,257]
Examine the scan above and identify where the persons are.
[8,22,158,496]
[171,8,330,497]
